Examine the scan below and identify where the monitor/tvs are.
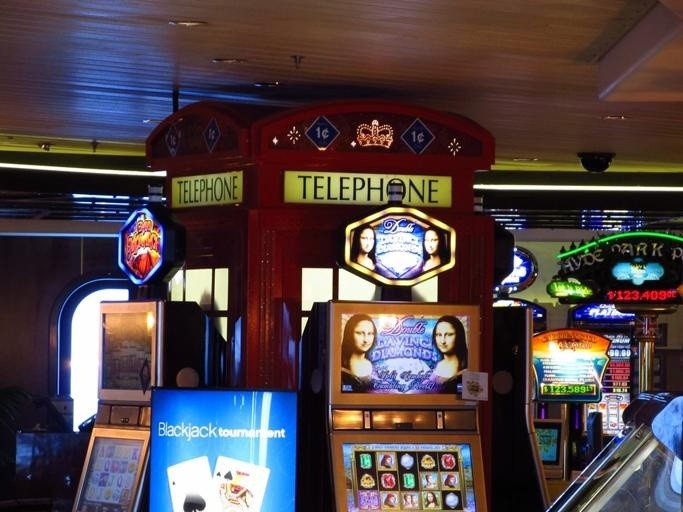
[99,301,208,403]
[70,427,150,512]
[534,420,568,480]
[329,431,488,512]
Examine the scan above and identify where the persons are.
[424,491,439,508]
[424,473,437,488]
[340,312,376,391]
[428,314,468,392]
[403,493,411,506]
[418,225,446,273]
[410,495,417,507]
[351,224,379,272]
